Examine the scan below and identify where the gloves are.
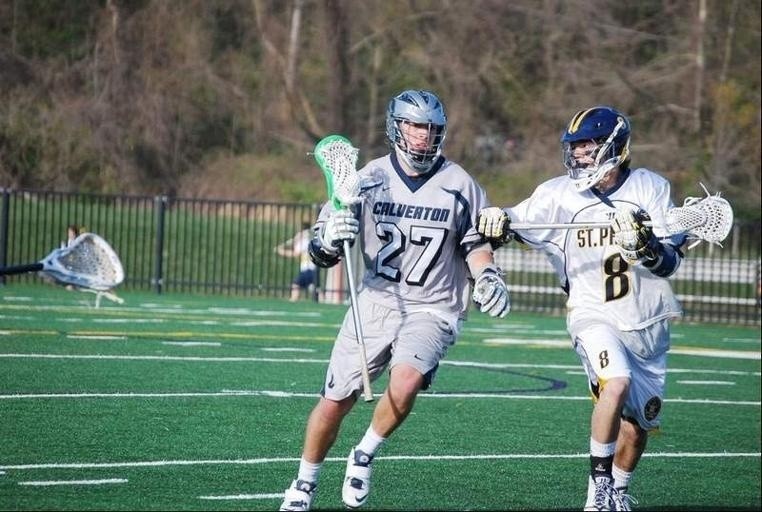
[471,264,512,318]
[609,209,657,266]
[475,206,512,241]
[322,209,361,252]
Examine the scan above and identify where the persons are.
[274,223,319,302]
[60,226,80,250]
[279,90,511,512]
[475,107,686,512]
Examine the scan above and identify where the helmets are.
[384,89,448,175]
[559,105,631,192]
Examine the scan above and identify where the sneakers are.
[612,485,640,512]
[583,473,615,512]
[341,445,375,509]
[279,476,318,512]
